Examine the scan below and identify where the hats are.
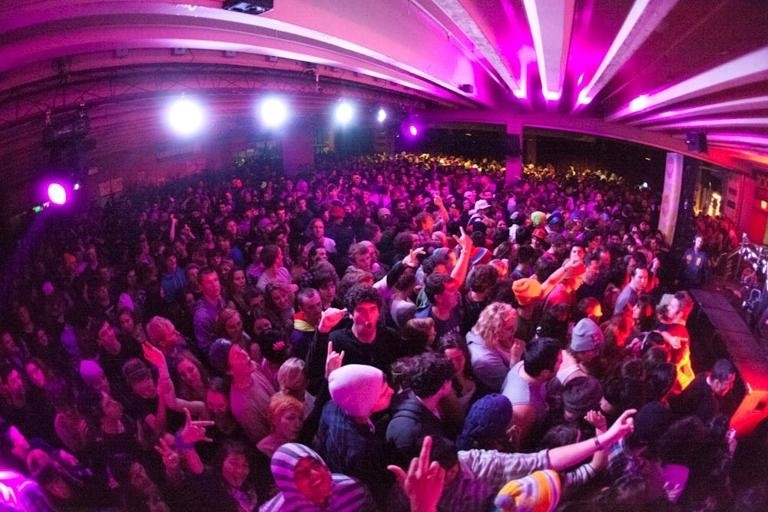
[208,338,232,373]
[465,393,512,435]
[468,200,492,215]
[562,258,587,279]
[434,247,450,258]
[563,376,601,412]
[571,318,604,351]
[328,364,385,415]
[470,246,508,279]
[512,278,544,306]
[531,210,561,225]
[494,469,561,512]
